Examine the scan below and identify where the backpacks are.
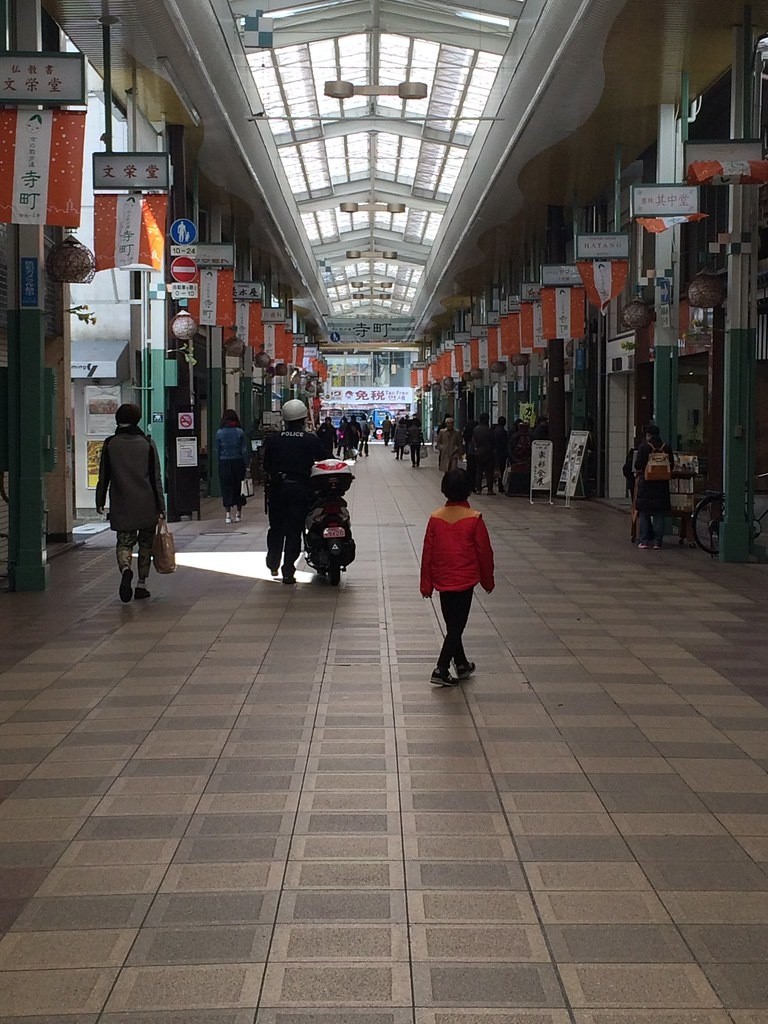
[644,441,672,481]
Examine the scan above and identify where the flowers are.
[681,320,729,339]
[166,340,197,365]
[621,342,656,351]
[225,367,245,375]
[43,305,96,325]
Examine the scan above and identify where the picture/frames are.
[86,439,110,489]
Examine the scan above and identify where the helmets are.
[282,399,308,422]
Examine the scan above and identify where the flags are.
[224,302,327,382]
[687,159,768,186]
[576,261,627,316]
[188,268,235,327]
[410,302,548,389]
[636,213,709,233]
[540,287,585,339]
[0,109,86,229]
[93,193,167,272]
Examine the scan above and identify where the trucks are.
[321,409,394,440]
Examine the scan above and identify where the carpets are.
[72,522,111,534]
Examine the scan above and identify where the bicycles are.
[690,473,768,555]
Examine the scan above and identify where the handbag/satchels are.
[152,519,176,574]
[403,444,410,454]
[240,470,254,498]
[420,445,428,458]
[391,443,397,453]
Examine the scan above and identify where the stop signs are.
[170,256,198,283]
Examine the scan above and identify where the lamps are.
[688,267,727,308]
[252,351,271,369]
[340,191,406,213]
[347,240,398,259]
[351,280,392,288]
[324,25,427,99]
[169,311,195,340]
[620,295,657,328]
[353,291,391,300]
[44,235,95,283]
[223,333,246,358]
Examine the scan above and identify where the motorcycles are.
[301,449,361,587]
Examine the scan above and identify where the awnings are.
[71,340,131,378]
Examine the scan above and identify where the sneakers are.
[430,668,460,686]
[457,662,476,679]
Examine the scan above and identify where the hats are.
[115,404,141,425]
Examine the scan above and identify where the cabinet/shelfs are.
[632,453,722,544]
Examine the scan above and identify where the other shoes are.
[119,569,134,604]
[134,587,151,599]
[282,573,296,584]
[638,542,661,549]
[265,557,280,575]
[235,511,242,522]
[226,512,232,524]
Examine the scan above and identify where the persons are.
[419,468,495,686]
[436,412,549,496]
[215,409,249,523]
[95,403,167,603]
[382,413,425,468]
[317,414,376,460]
[262,399,326,584]
[623,425,674,548]
